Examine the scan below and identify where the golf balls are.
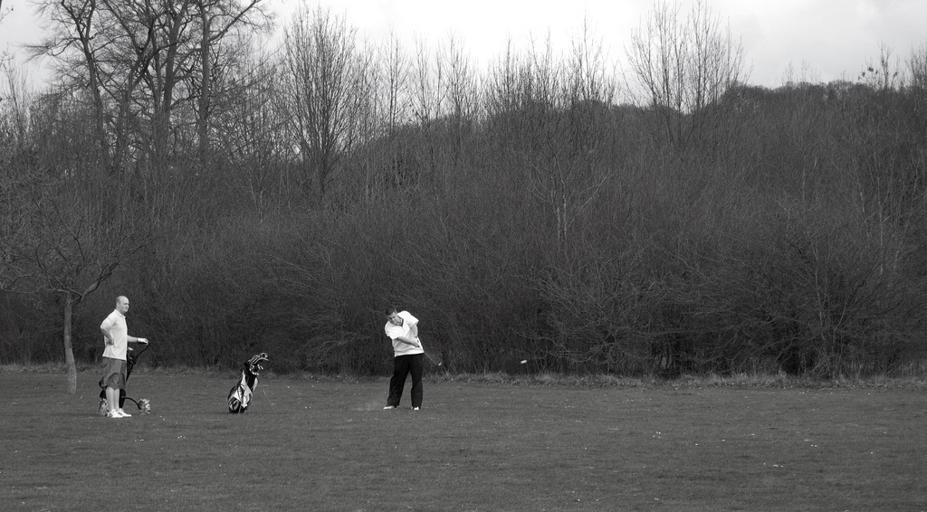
[522,359,527,364]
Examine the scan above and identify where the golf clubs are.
[249,353,268,374]
[418,346,441,367]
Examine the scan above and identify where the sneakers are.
[107,408,132,419]
[383,406,400,410]
[410,405,419,411]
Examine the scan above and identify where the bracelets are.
[413,335,417,339]
[136,337,141,342]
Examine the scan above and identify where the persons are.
[383,304,426,411]
[99,295,150,419]
